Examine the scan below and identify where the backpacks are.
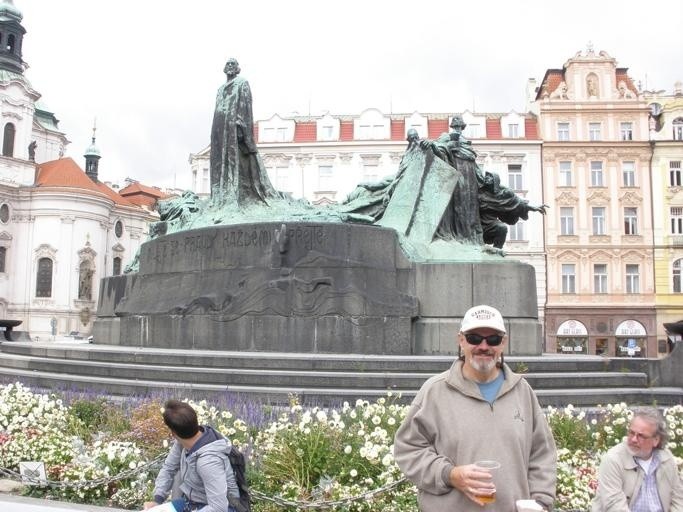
[197,446,250,512]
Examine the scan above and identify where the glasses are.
[464,334,502,346]
[629,429,655,440]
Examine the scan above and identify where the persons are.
[206,57,277,209]
[348,110,549,247]
[393,304,558,512]
[145,399,248,512]
[587,405,682,512]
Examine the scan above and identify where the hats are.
[461,305,506,333]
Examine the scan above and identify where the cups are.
[474,459,500,503]
